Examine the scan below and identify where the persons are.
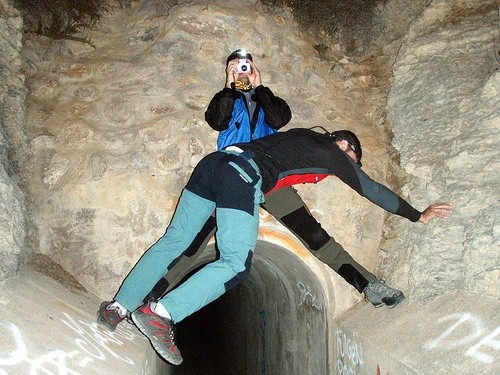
[97,126,454,365]
[125,49,406,325]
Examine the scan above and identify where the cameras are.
[235,59,251,74]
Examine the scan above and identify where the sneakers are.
[130,298,183,367]
[363,277,406,310]
[96,298,125,334]
